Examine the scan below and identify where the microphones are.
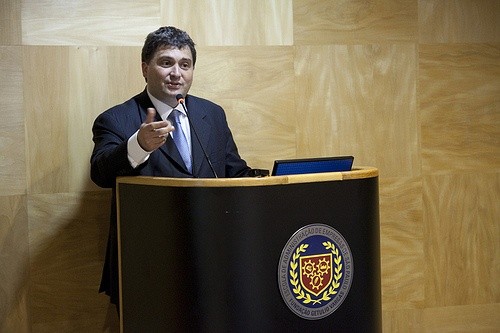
[176,94,219,178]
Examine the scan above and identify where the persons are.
[90,25,255,320]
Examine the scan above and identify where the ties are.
[170,110,192,175]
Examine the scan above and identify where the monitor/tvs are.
[272,155,354,176]
